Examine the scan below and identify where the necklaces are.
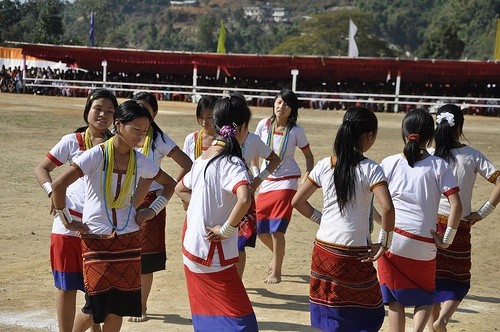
[103,137,138,232]
[267,115,290,174]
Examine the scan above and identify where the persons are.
[372,110,463,332]
[127,92,193,324]
[426,104,500,332]
[250,88,314,283]
[34,88,111,332]
[176,94,258,332]
[52,101,177,332]
[239,129,282,282]
[182,96,221,162]
[291,108,395,332]
[0,64,500,107]
